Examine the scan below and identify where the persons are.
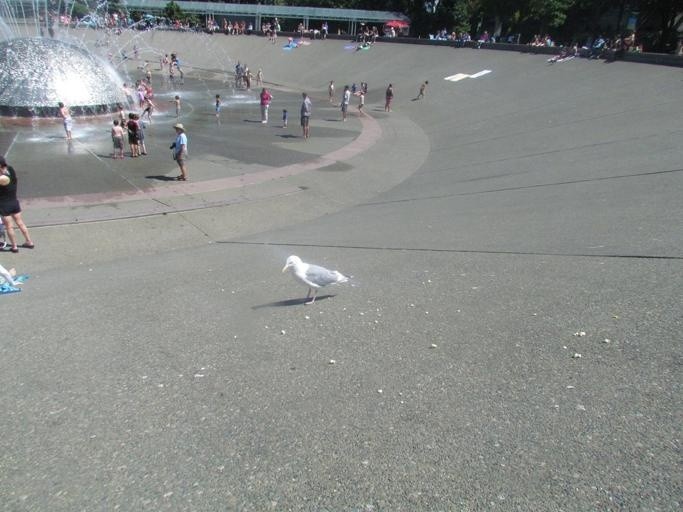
[260,87,273,124]
[173,123,189,181]
[283,108,288,128]
[301,92,312,137]
[417,27,636,64]
[341,80,369,122]
[1,155,34,253]
[33,8,203,38]
[95,39,185,123]
[215,94,222,118]
[233,59,263,91]
[58,102,73,139]
[385,84,394,112]
[203,17,410,51]
[111,105,148,160]
[418,80,428,99]
[328,80,337,102]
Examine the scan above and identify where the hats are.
[173,123,186,132]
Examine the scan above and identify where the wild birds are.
[280,254,349,305]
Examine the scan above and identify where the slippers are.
[9,244,34,253]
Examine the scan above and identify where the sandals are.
[177,174,186,181]
